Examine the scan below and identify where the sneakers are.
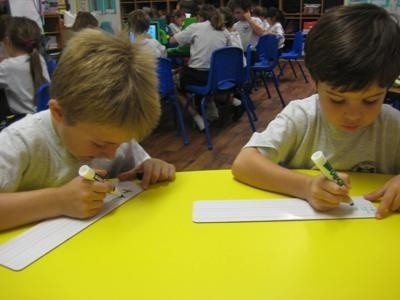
[198,120,209,131]
[232,99,241,106]
[205,109,218,122]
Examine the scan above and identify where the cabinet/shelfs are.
[120,0,348,62]
[42,12,66,65]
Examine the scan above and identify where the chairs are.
[158,31,309,151]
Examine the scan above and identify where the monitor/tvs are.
[128,21,160,47]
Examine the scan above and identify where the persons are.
[232,3,400,219]
[1,0,285,131]
[0,28,175,232]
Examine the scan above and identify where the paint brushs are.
[79,165,124,198]
[311,151,354,206]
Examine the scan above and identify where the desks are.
[0,168,400,299]
